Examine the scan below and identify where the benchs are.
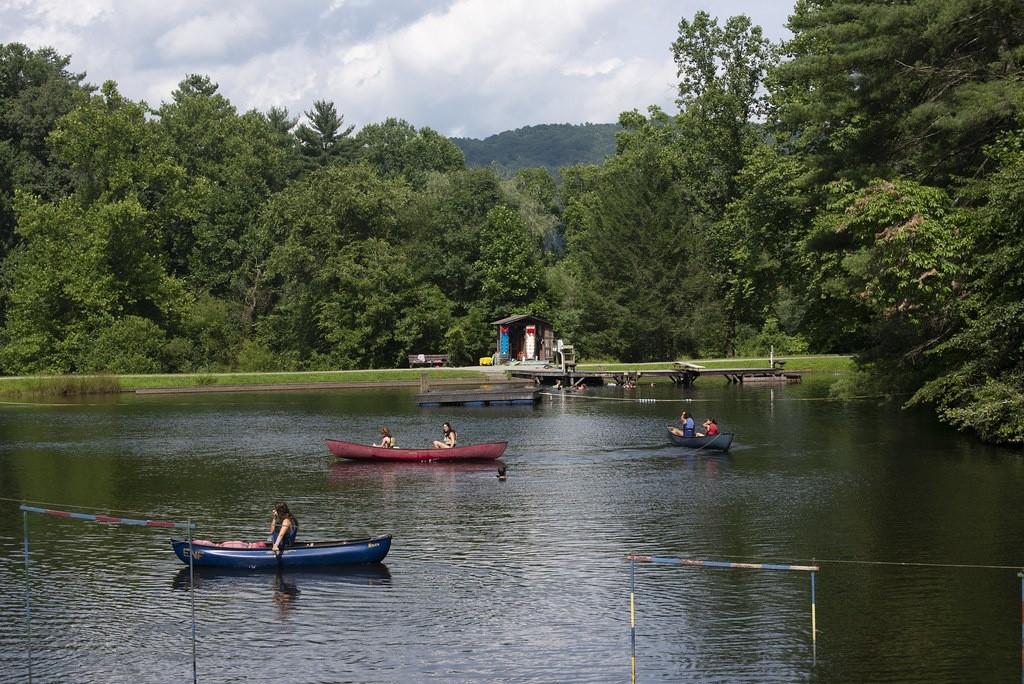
[408,354,453,369]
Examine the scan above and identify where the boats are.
[326,438,508,461]
[665,424,734,450]
[169,533,392,573]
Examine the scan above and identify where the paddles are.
[274,551,284,584]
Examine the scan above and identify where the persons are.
[434,422,457,449]
[373,427,395,448]
[696,417,718,437]
[270,503,299,551]
[672,411,695,437]
